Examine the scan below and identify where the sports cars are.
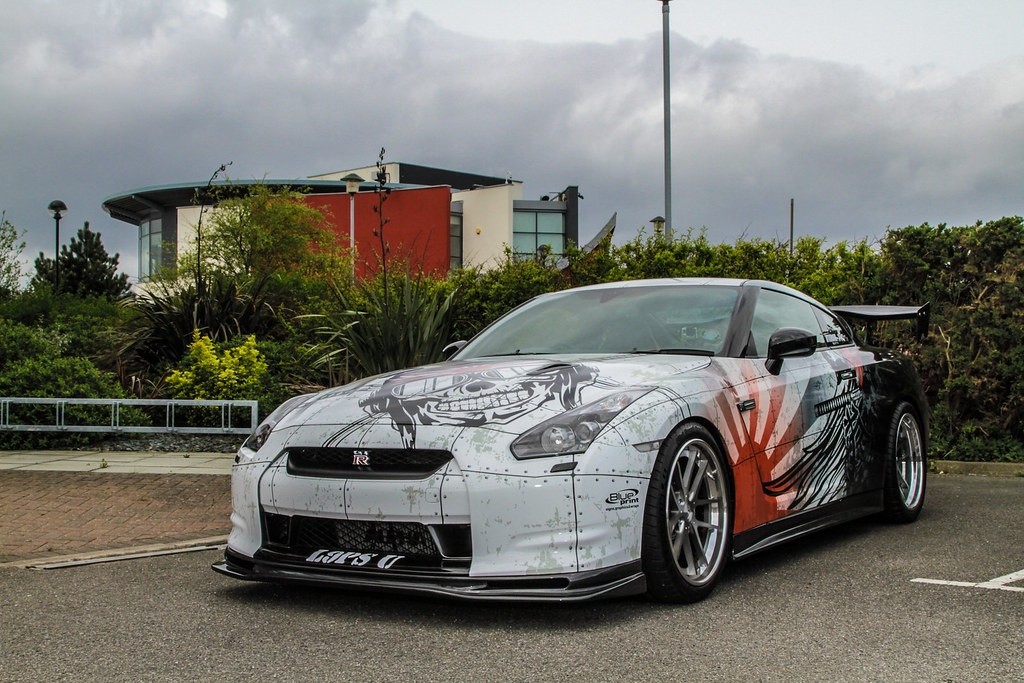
[211,277,931,606]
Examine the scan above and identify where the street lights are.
[340,173,365,290]
[46,199,68,295]
